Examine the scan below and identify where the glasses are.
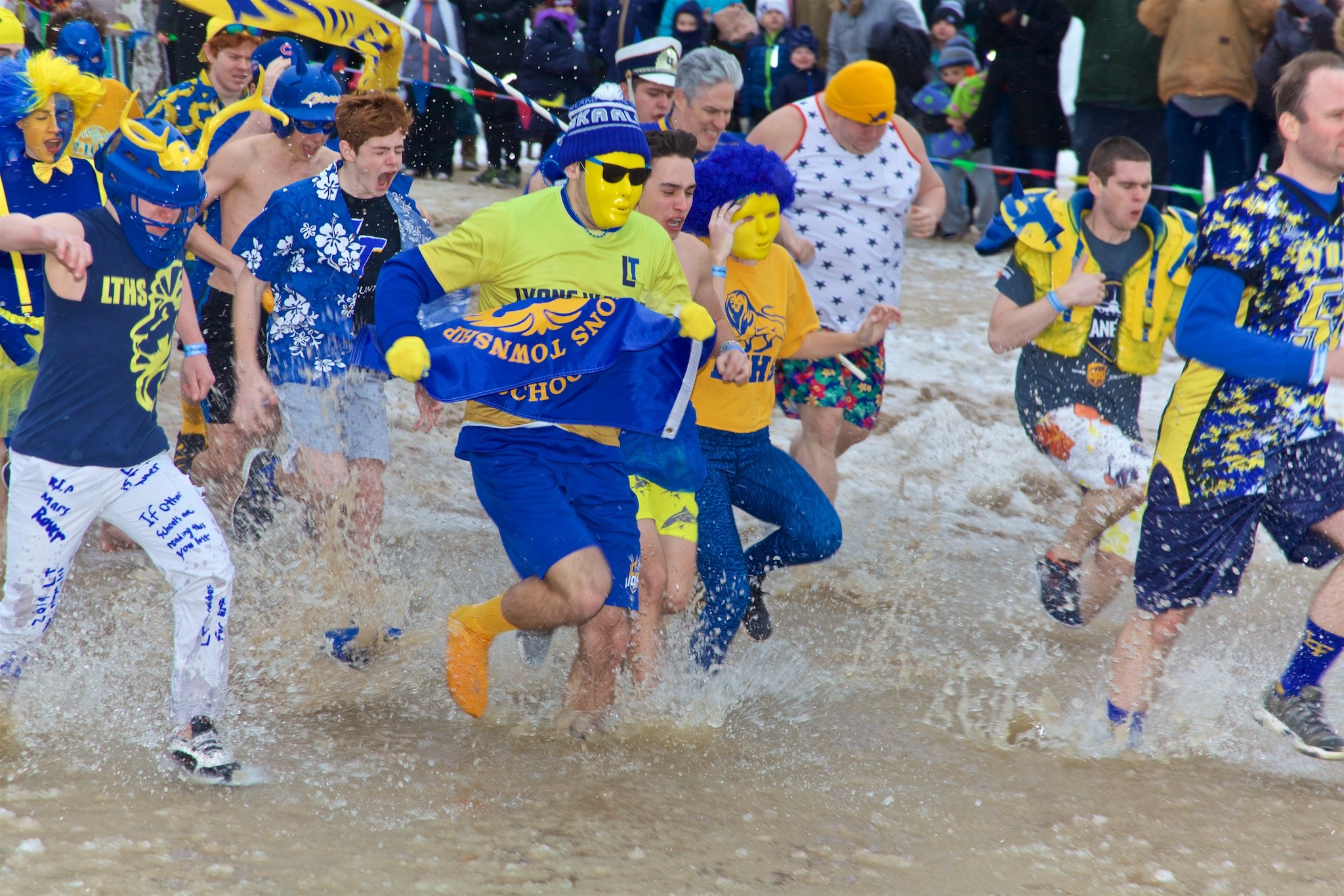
[587,157,652,185]
[225,23,261,36]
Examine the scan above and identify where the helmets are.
[270,47,341,139]
[93,60,288,270]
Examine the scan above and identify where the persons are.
[377,82,714,721]
[229,91,444,666]
[683,144,902,688]
[0,0,340,552]
[378,0,826,190]
[1109,49,1344,761]
[526,47,744,199]
[0,115,243,778]
[626,128,752,686]
[184,51,344,540]
[750,60,947,503]
[975,137,1196,622]
[725,0,1344,241]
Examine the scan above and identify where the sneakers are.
[1253,680,1344,762]
[232,448,279,541]
[173,715,237,778]
[1038,552,1083,627]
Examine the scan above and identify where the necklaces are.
[542,159,608,238]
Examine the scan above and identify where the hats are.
[936,34,981,71]
[755,0,790,25]
[250,37,311,76]
[615,37,683,87]
[557,80,650,169]
[673,0,702,40]
[788,24,819,52]
[0,7,25,45]
[198,15,264,62]
[824,60,895,124]
[930,0,966,26]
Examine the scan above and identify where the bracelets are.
[712,266,726,277]
[1309,353,1326,386]
[720,340,744,354]
[726,346,746,354]
[1047,292,1066,312]
[183,343,207,357]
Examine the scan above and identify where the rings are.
[719,215,723,217]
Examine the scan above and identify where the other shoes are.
[743,574,772,642]
[405,160,522,189]
